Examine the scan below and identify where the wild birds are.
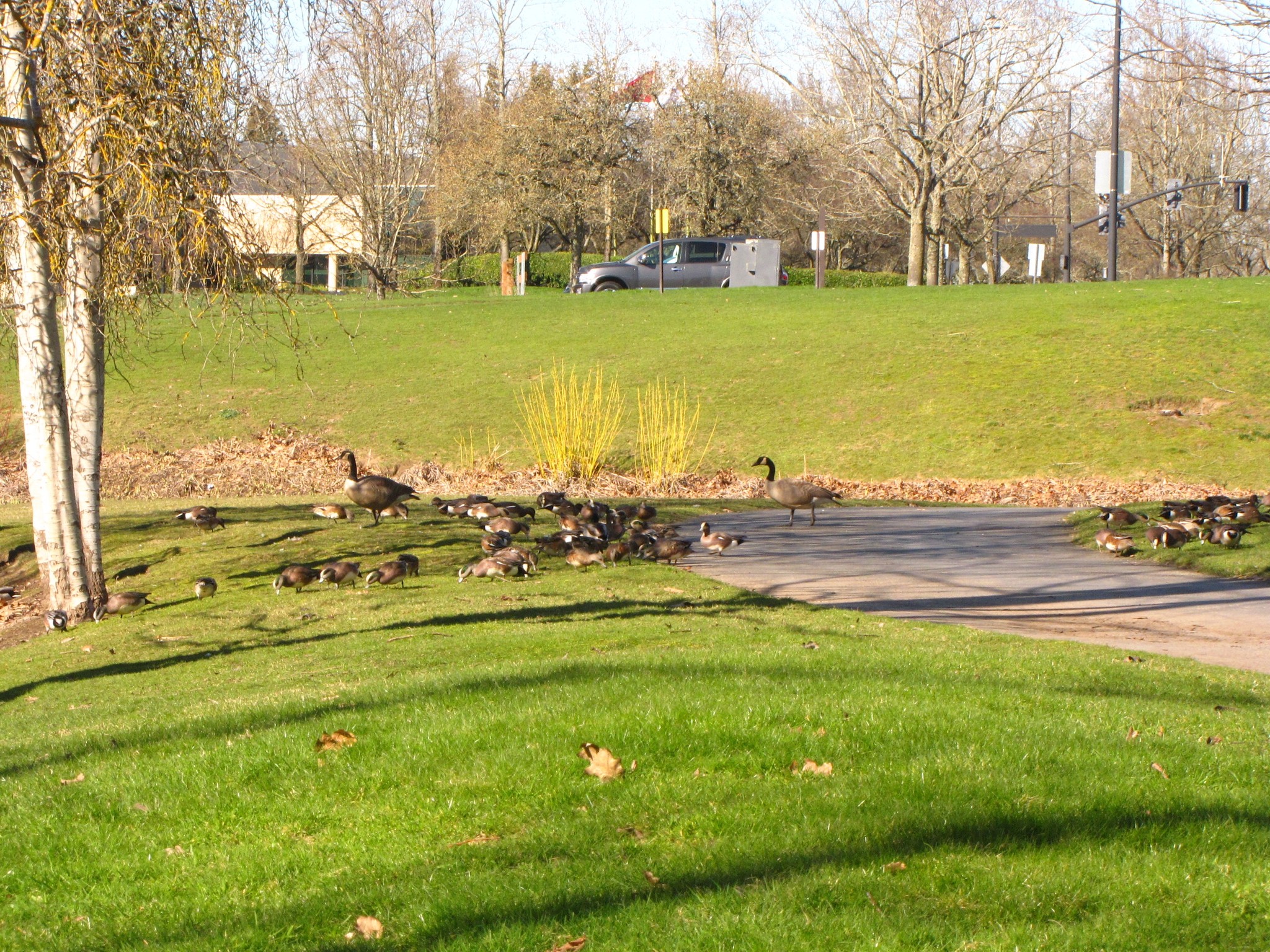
[307,450,422,527]
[272,562,364,595]
[1095,504,1151,533]
[365,554,420,591]
[93,591,156,624]
[751,456,843,527]
[169,506,226,534]
[0,587,22,605]
[1095,528,1139,557]
[194,577,217,601]
[39,609,69,637]
[1146,491,1270,552]
[427,492,693,584]
[699,522,748,557]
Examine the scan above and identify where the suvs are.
[565,235,787,294]
